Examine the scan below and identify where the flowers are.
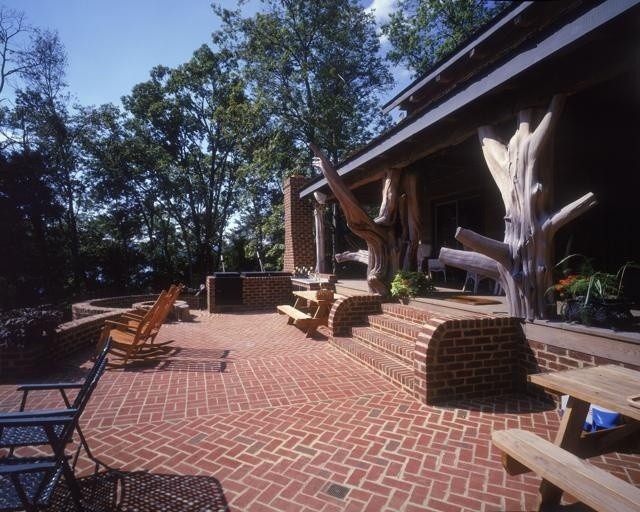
[543,272,589,300]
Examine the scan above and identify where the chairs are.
[0,335,112,512]
[461,244,504,296]
[89,283,186,370]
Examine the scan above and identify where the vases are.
[564,299,583,323]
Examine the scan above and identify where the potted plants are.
[554,253,640,331]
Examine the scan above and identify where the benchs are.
[490,428,640,512]
[276,304,312,321]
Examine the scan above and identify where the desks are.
[525,363,640,512]
[291,278,320,307]
[286,289,346,339]
